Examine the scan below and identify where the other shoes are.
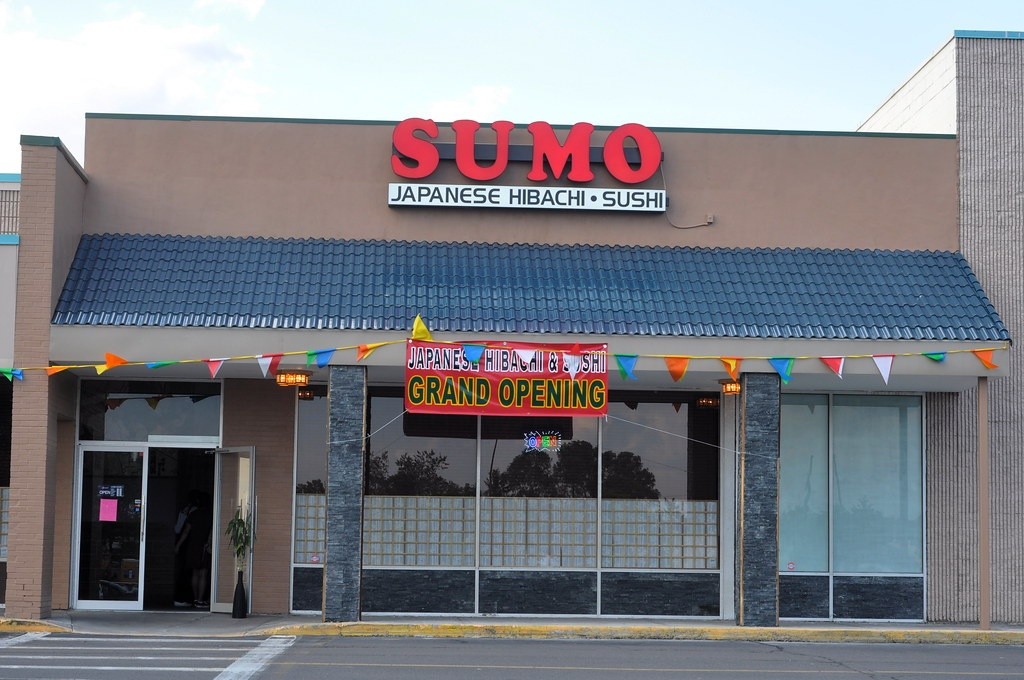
[194,599,209,608]
[174,600,193,607]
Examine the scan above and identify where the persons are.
[173,489,212,609]
